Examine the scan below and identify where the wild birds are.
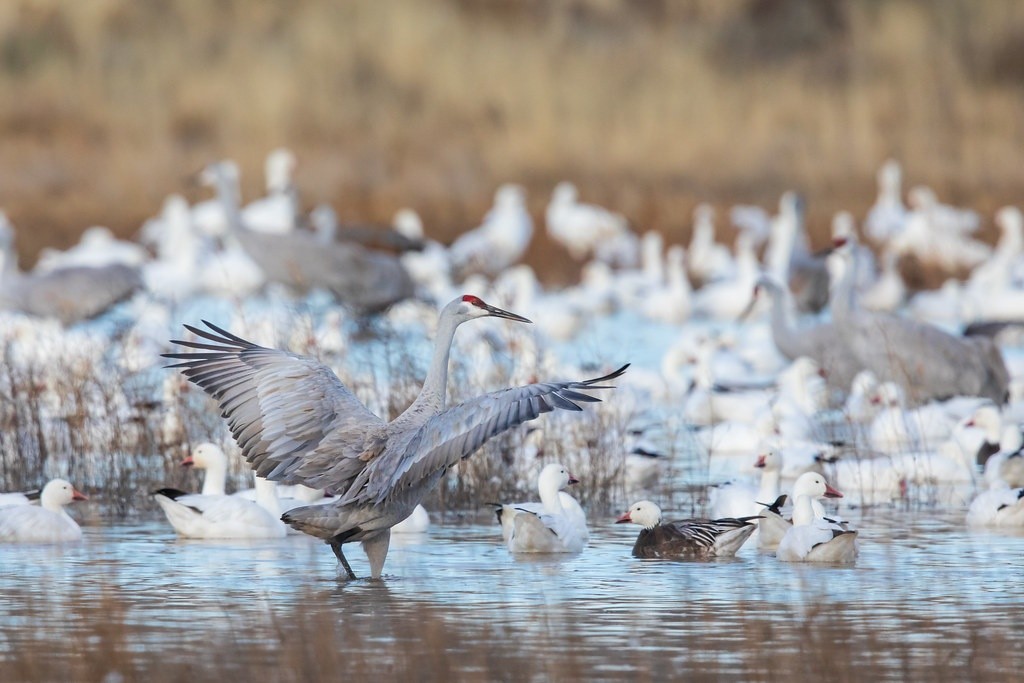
[158,295,632,581]
[150,470,287,539]
[483,462,590,553]
[755,493,849,550]
[1,148,1024,534]
[0,477,87,541]
[616,500,767,562]
[776,471,859,562]
[180,441,324,504]
